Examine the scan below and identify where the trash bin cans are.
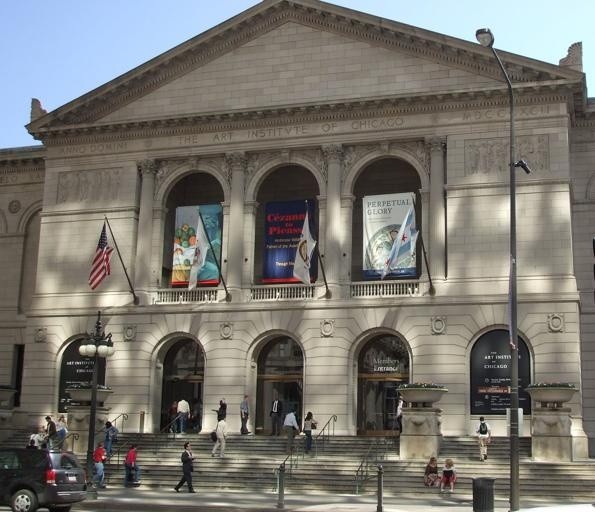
[472,477,496,512]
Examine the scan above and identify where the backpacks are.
[480,423,487,434]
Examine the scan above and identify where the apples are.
[175,224,195,266]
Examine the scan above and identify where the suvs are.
[0,448,87,512]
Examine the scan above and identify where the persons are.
[440,458,457,492]
[424,456,439,486]
[238,393,252,437]
[89,441,143,489]
[169,397,202,434]
[173,441,196,493]
[269,393,318,456]
[474,416,492,462]
[102,420,120,454]
[211,397,228,423]
[210,416,230,459]
[24,413,68,451]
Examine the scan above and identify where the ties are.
[274,403,276,411]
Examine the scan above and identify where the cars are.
[369,413,401,433]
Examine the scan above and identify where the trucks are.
[281,400,301,433]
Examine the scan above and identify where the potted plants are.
[64,380,113,402]
[524,383,579,403]
[396,380,448,402]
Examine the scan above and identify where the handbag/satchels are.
[211,432,217,442]
[311,423,316,429]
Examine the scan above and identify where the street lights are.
[79,307,118,500]
[475,25,520,512]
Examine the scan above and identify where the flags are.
[187,215,211,291]
[380,203,420,281]
[87,219,115,291]
[292,208,318,286]
